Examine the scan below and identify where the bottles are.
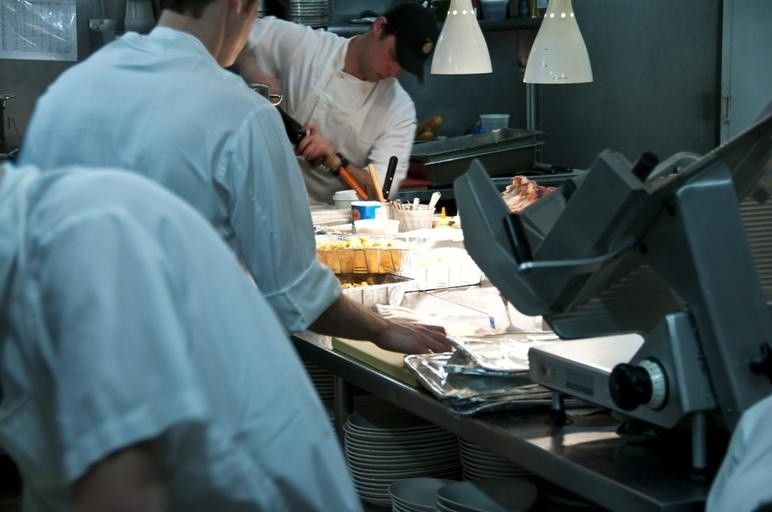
[275,104,326,169]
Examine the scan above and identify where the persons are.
[0,157,364,512]
[18,0,455,357]
[234,0,438,207]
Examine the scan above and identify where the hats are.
[383,4,439,76]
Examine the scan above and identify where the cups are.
[125,1,154,29]
[332,191,435,236]
[480,114,510,131]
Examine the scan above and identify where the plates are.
[301,359,540,512]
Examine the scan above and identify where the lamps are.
[430,0,595,86]
